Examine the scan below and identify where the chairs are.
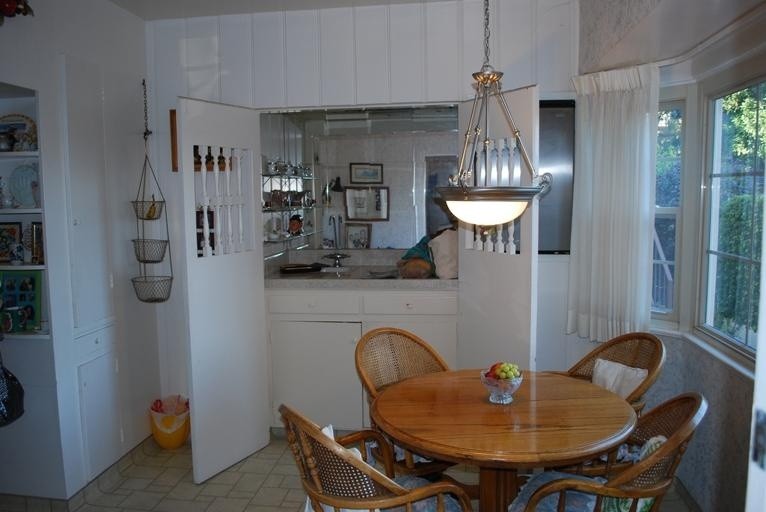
[507,390,710,512]
[544,332,667,409]
[354,326,459,477]
[278,402,475,512]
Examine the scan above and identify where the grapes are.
[496,362,519,379]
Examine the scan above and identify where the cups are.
[3,306,28,334]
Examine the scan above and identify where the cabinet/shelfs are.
[0,96,51,340]
[77,349,124,478]
[65,53,119,340]
[269,290,459,428]
[262,175,324,243]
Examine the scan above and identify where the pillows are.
[591,357,649,400]
[589,436,669,511]
[306,423,381,512]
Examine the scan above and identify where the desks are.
[369,368,638,512]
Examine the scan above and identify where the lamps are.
[434,0,554,227]
[322,176,346,197]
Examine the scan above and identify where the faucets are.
[329,215,339,255]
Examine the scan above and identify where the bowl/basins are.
[480,369,523,405]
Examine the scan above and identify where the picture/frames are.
[0,270,41,330]
[349,162,383,184]
[345,222,372,249]
[343,186,390,222]
[169,109,232,172]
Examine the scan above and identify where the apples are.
[490,362,503,373]
[484,371,499,386]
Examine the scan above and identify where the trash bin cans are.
[149,398,190,449]
